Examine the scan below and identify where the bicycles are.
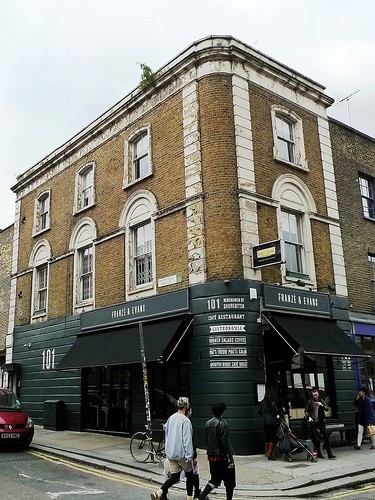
[129,422,167,469]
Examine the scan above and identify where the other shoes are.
[369,445,375,450]
[194,497,199,500]
[317,454,325,458]
[328,454,336,459]
[353,445,360,450]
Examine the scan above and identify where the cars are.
[0,388,35,450]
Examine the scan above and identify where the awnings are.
[54,314,194,369]
[262,311,370,357]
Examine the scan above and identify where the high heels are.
[268,456,277,461]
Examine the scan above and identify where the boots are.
[151,489,163,500]
[160,489,170,500]
[194,489,209,500]
[187,496,193,500]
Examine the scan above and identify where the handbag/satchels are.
[280,438,291,454]
[309,421,323,442]
[367,425,375,437]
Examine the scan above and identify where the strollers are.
[276,415,319,463]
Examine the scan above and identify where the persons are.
[309,387,330,407]
[289,389,304,408]
[257,388,283,460]
[305,389,336,458]
[288,427,318,456]
[353,386,375,450]
[150,397,209,500]
[193,401,236,500]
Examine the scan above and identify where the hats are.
[177,397,189,408]
